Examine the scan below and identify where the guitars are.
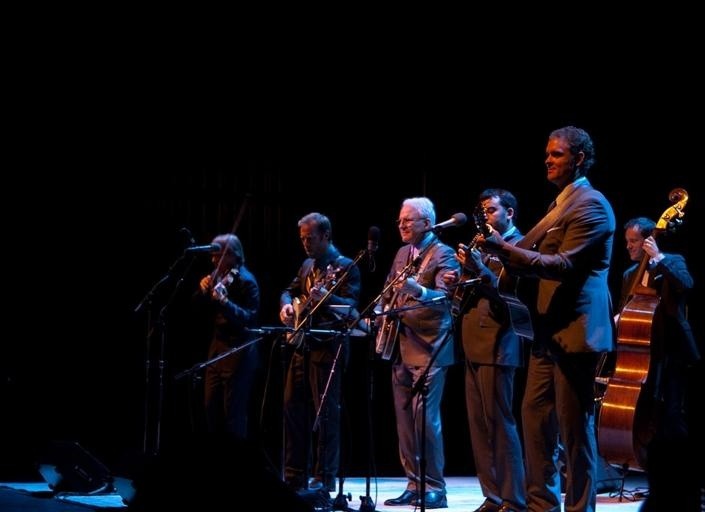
[472,202,535,341]
[286,265,345,350]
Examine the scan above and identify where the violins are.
[204,266,245,298]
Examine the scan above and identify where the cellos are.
[594,185,689,502]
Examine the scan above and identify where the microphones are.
[187,242,222,255]
[362,226,381,271]
[419,212,468,234]
[450,273,492,291]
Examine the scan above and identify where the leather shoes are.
[470,496,499,512]
[409,490,448,509]
[296,476,330,497]
[384,489,416,506]
[496,501,519,512]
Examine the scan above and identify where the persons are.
[370,195,462,510]
[276,211,362,499]
[608,217,701,510]
[440,189,535,510]
[478,125,618,510]
[191,234,261,494]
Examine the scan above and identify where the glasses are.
[211,250,231,258]
[397,217,426,222]
